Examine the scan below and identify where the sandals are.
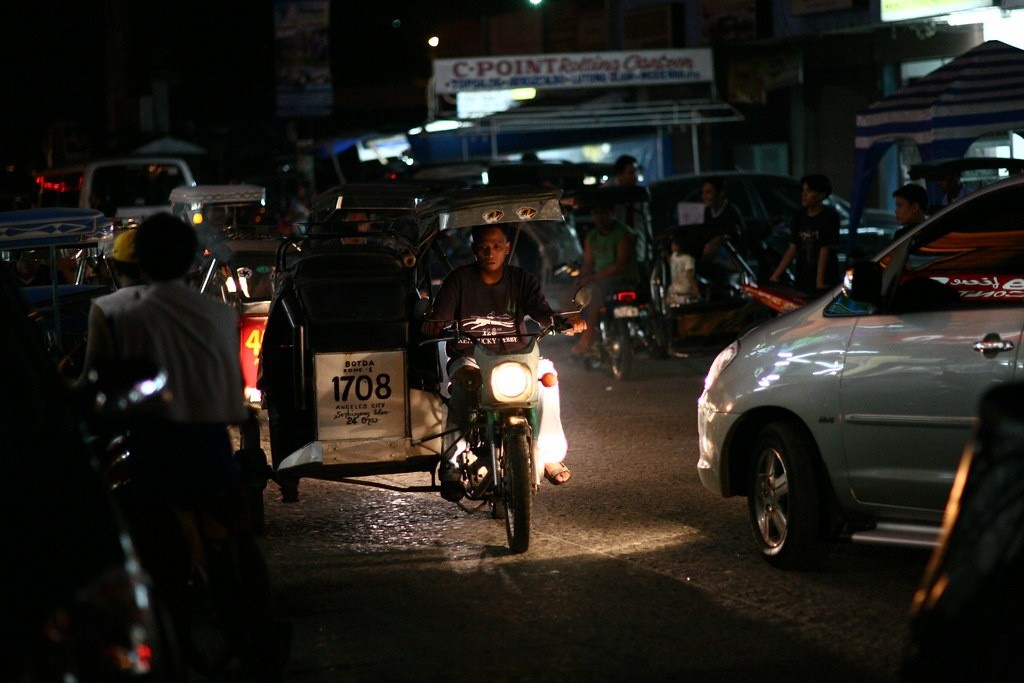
[543,460,572,485]
[439,481,467,503]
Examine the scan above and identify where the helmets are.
[110,229,138,262]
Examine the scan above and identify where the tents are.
[846,41,1024,265]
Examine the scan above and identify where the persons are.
[769,175,841,299]
[6,248,72,287]
[881,183,944,313]
[78,211,246,534]
[941,175,974,205]
[696,176,746,261]
[204,205,228,232]
[323,210,383,245]
[275,185,325,232]
[667,233,699,305]
[96,229,142,286]
[558,202,635,354]
[603,155,655,297]
[421,224,587,502]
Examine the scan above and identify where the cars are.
[693,171,1023,574]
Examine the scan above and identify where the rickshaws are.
[0,149,319,683]
[650,175,920,358]
[257,166,595,555]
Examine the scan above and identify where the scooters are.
[557,258,648,380]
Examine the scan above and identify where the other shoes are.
[569,330,594,357]
